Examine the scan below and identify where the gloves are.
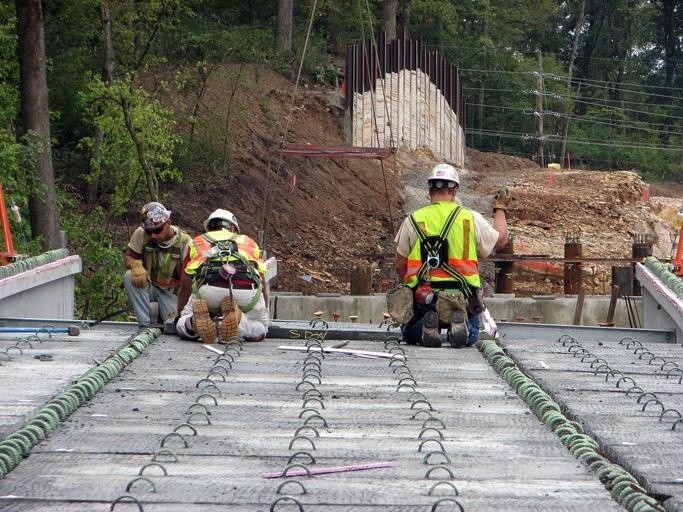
[130,260,152,289]
[493,184,513,213]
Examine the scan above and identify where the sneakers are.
[450,311,468,346]
[220,295,238,341]
[192,299,217,344]
[423,310,443,348]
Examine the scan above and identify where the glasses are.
[144,226,164,235]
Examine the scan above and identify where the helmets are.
[140,202,172,230]
[427,164,460,186]
[202,209,240,233]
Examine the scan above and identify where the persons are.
[172,207,269,341]
[392,163,512,348]
[120,200,195,330]
